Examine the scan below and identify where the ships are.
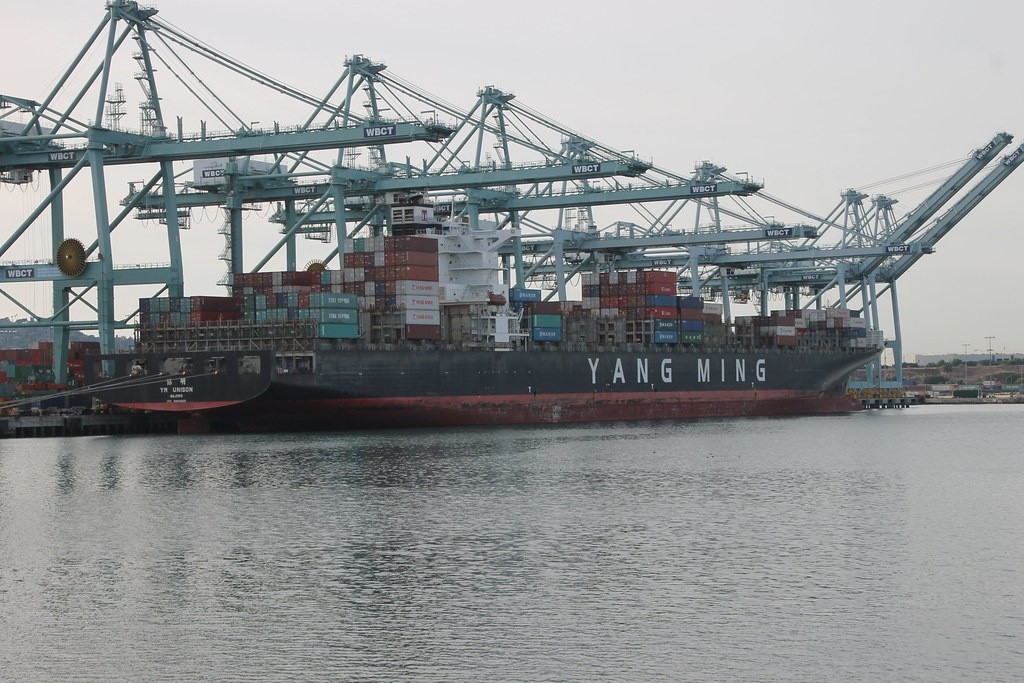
[76,190,888,436]
